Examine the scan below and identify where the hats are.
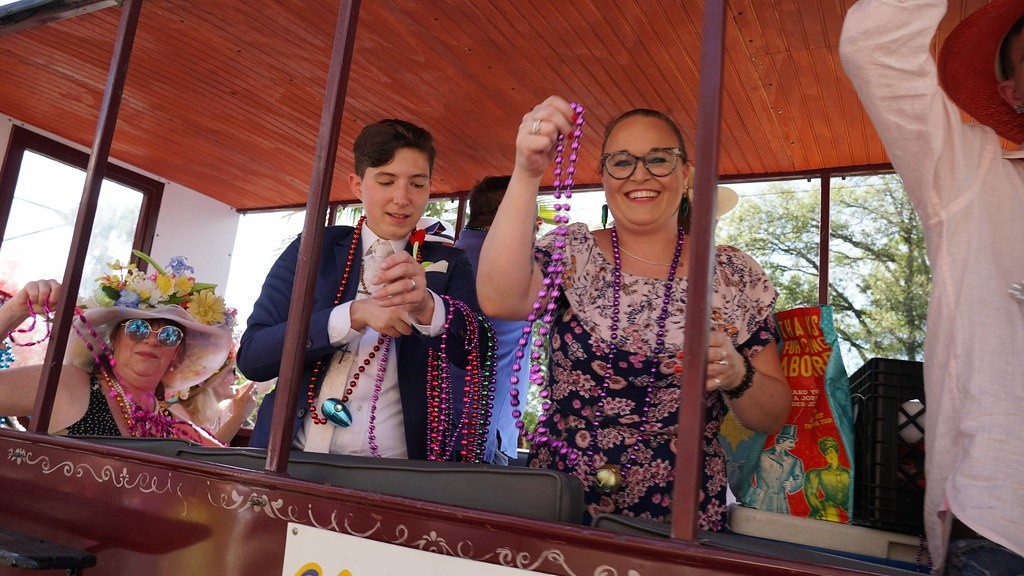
[69,251,233,392]
[686,169,740,217]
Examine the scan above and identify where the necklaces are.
[427,291,498,461]
[587,224,684,492]
[510,102,585,466]
[308,213,422,424]
[466,225,487,231]
[618,246,671,266]
[1,296,222,443]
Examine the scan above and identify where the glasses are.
[600,147,682,180]
[122,318,184,348]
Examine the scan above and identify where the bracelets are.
[723,355,754,399]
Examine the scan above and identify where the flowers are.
[85,248,236,328]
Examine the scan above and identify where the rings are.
[715,351,728,364]
[714,379,721,387]
[409,278,416,291]
[533,118,542,135]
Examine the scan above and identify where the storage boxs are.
[851,358,925,536]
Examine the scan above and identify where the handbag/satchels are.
[720,306,855,527]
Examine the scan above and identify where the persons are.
[454,174,543,461]
[1,257,230,446]
[170,353,276,444]
[235,120,487,461]
[838,0,1024,576]
[476,95,791,535]
